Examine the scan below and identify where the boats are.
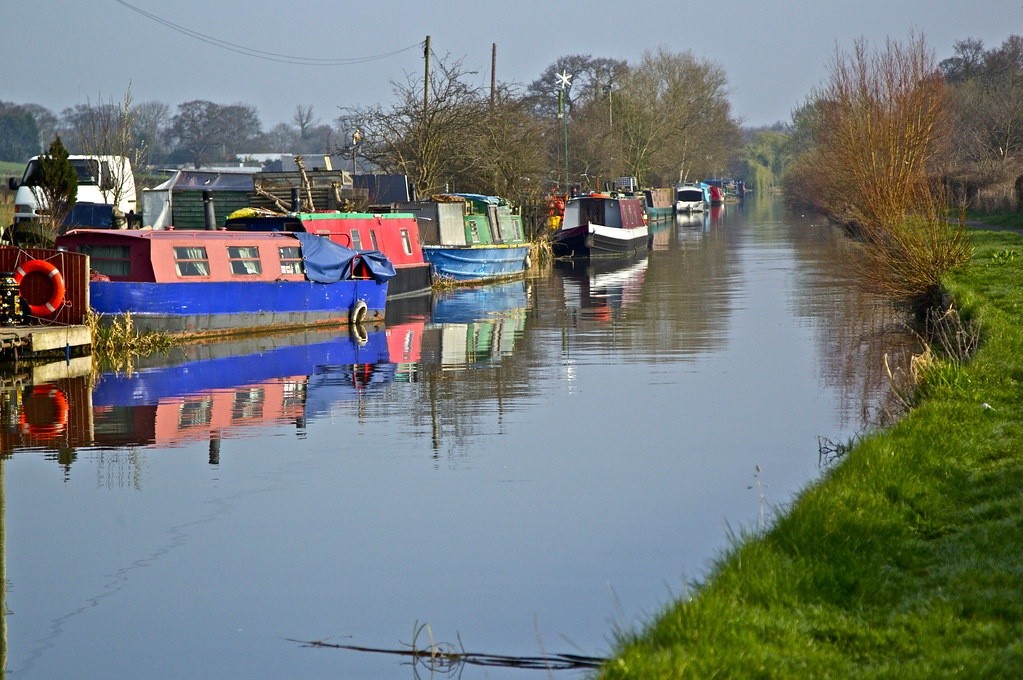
[420,242,534,288]
[546,248,653,324]
[92,319,430,464]
[641,187,673,222]
[674,185,705,213]
[224,187,434,306]
[49,185,398,347]
[549,181,652,260]
[701,179,738,203]
[676,212,703,228]
[650,217,673,251]
[420,279,535,457]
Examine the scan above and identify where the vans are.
[9,154,137,231]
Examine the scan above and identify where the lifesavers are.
[13,258,66,318]
[350,300,368,324]
[18,384,70,441]
[351,324,369,344]
